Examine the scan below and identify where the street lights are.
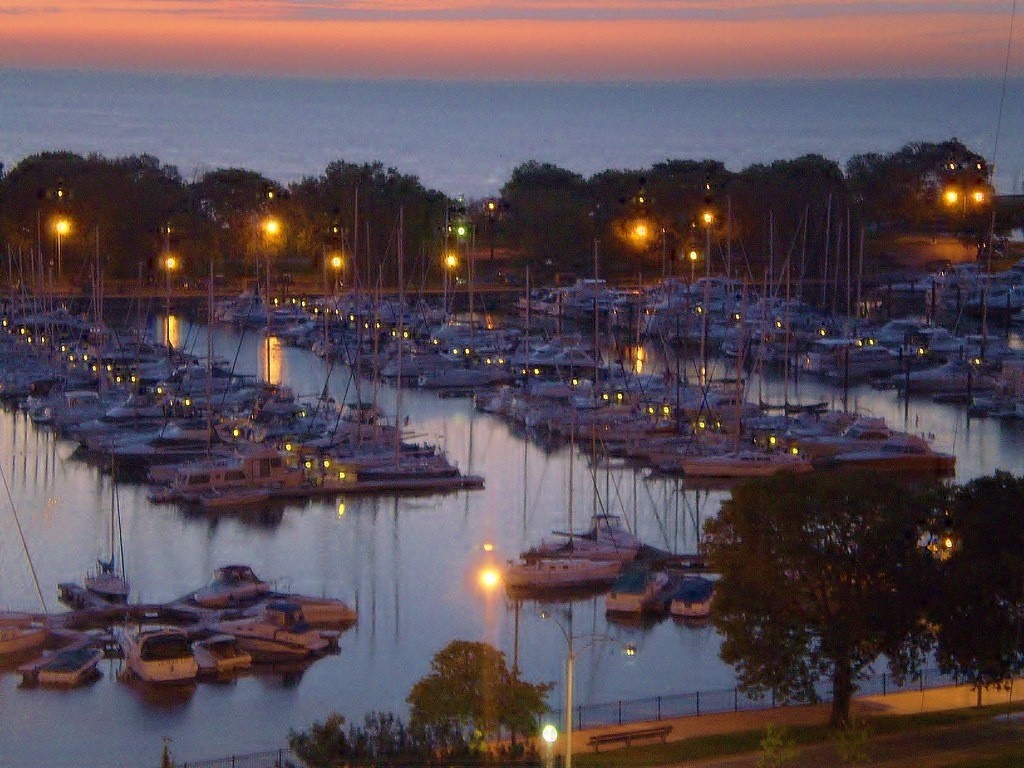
[945,189,968,220]
[974,191,997,360]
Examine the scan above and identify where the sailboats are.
[0,226,1023,690]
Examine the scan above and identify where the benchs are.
[586,724,671,751]
